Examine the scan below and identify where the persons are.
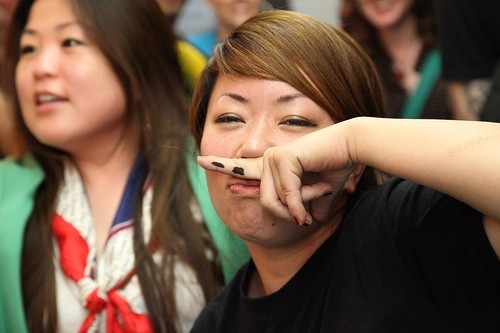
[0,0,252,333]
[191,10,500,333]
[156,0,500,119]
